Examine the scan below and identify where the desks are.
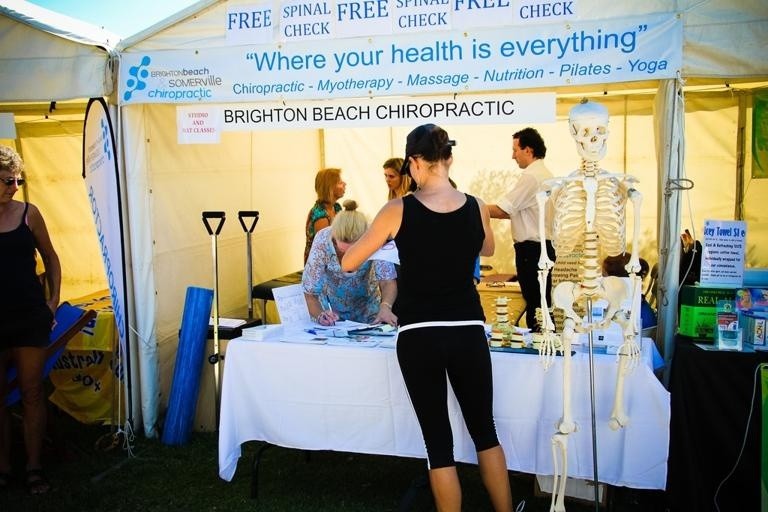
[663,330,767,512]
[252,269,305,328]
[45,289,131,449]
[209,316,674,506]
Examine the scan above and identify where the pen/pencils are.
[328,302,335,325]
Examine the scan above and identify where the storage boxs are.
[739,270,767,316]
[471,281,530,329]
[676,281,741,341]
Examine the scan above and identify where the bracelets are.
[379,301,392,309]
[312,309,323,322]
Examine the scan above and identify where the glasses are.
[0,177,26,186]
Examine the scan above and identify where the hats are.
[399,124,455,176]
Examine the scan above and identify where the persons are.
[487,128,557,333]
[302,167,347,267]
[341,123,514,512]
[0,145,62,494]
[602,252,657,331]
[382,157,411,199]
[301,198,398,328]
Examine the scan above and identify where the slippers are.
[0,474,8,491]
[23,469,52,496]
